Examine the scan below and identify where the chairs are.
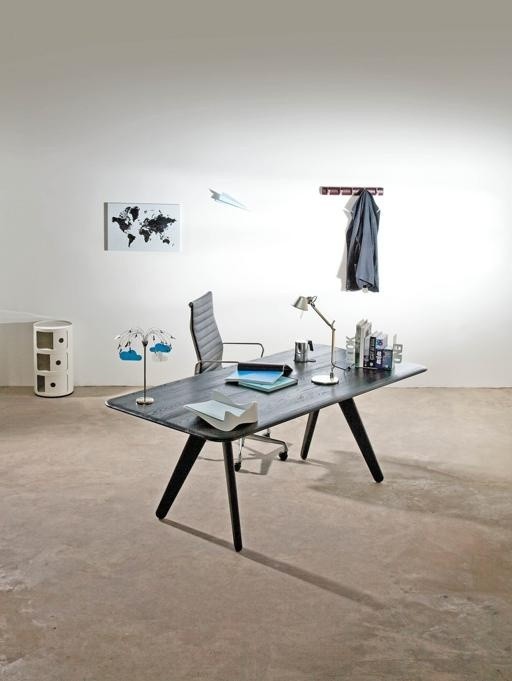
[188,292,287,473]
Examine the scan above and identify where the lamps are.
[114,325,177,406]
[291,295,340,385]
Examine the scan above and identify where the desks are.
[108,343,427,552]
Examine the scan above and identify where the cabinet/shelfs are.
[33,319,74,398]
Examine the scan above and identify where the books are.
[355,318,395,372]
[226,362,294,385]
[237,377,299,396]
